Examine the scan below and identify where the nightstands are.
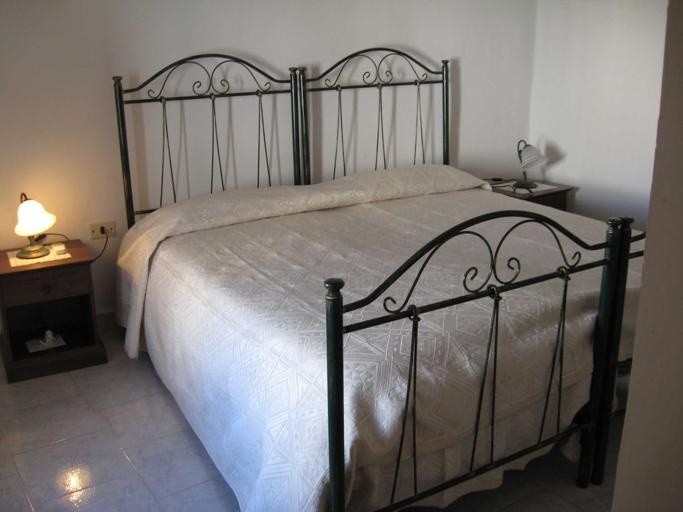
[0,239,108,383]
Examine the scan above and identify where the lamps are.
[15,193,56,259]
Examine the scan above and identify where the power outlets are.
[89,220,117,240]
